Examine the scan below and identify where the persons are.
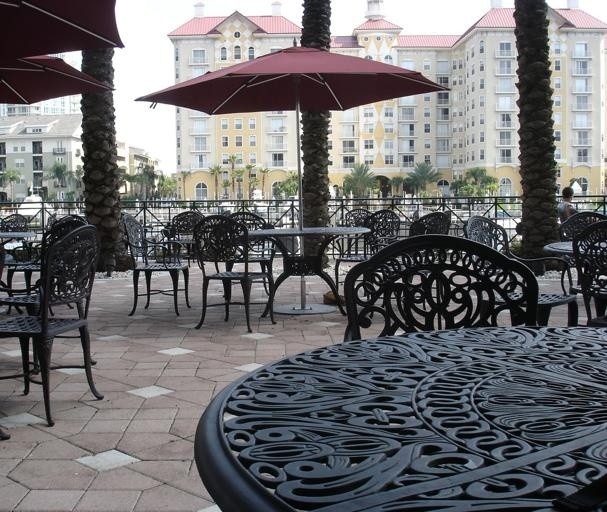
[560,187,579,225]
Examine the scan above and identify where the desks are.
[193,324,606,512]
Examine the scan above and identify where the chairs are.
[1,211,105,442]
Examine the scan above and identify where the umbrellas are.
[0,0,126,60]
[0,53,115,104]
[134,36,451,316]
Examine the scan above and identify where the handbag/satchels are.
[323,291,346,306]
